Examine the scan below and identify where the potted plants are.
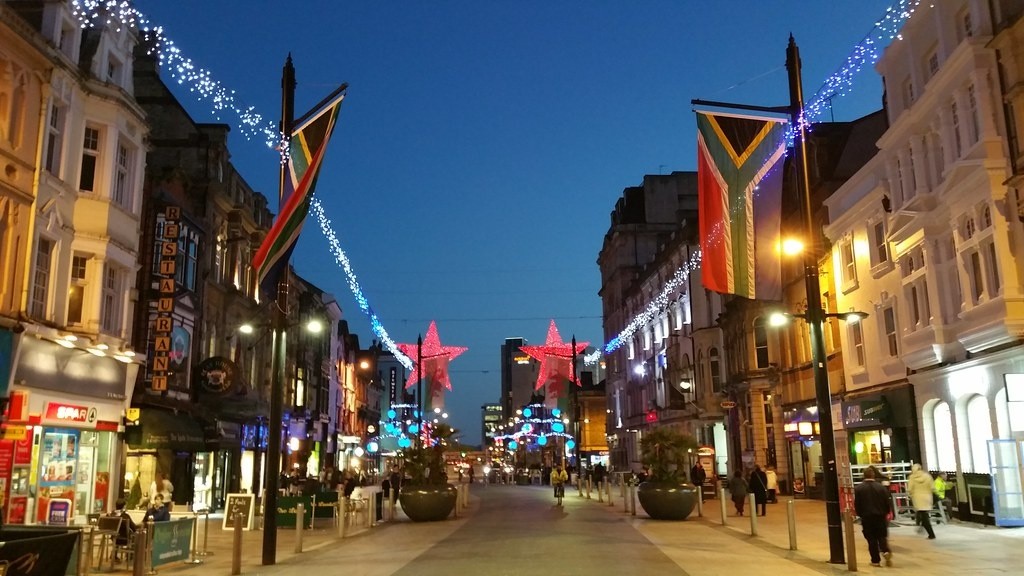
[637,425,699,520]
[399,424,461,521]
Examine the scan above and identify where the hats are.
[557,462,562,466]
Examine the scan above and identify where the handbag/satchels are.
[886,507,893,521]
[764,490,771,499]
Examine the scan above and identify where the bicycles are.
[555,478,566,506]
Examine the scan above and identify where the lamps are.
[8,311,25,334]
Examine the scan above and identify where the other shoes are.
[736,512,738,514]
[740,512,743,516]
[883,552,892,566]
[869,563,880,566]
[772,498,778,503]
[928,536,935,538]
[110,555,120,561]
[702,500,706,504]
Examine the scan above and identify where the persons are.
[529,461,778,517]
[280,464,474,509]
[108,476,174,562]
[856,463,954,565]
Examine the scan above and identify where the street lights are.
[563,418,590,490]
[236,316,325,567]
[751,309,872,566]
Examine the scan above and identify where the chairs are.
[85,514,139,573]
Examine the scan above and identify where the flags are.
[251,95,344,302]
[693,110,786,303]
